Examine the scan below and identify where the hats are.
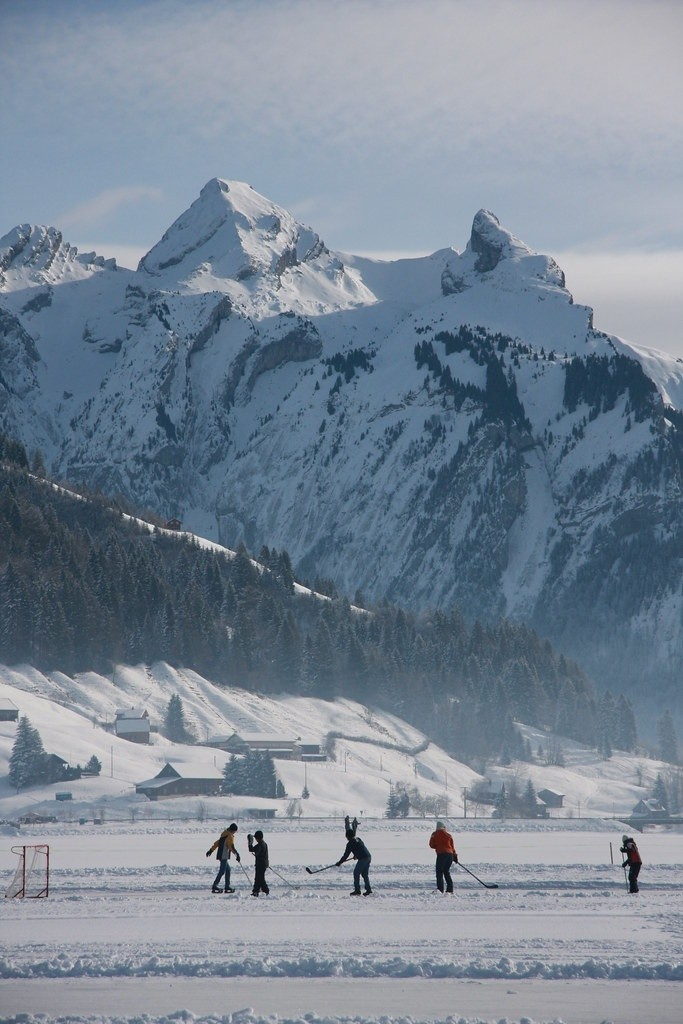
[345,828,354,837]
[436,821,444,828]
[254,831,263,839]
[622,835,628,842]
[230,823,238,830]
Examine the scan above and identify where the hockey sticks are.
[621,851,629,893]
[251,852,301,890]
[456,861,499,889]
[305,858,354,875]
[233,850,253,887]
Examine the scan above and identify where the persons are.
[344,815,350,838]
[429,821,459,895]
[352,816,361,836]
[205,823,240,893]
[620,834,643,894]
[336,829,373,896]
[247,830,270,897]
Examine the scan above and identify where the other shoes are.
[251,891,258,896]
[211,886,223,893]
[225,888,235,893]
[363,890,372,896]
[349,891,361,896]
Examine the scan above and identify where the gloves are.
[236,855,241,863]
[622,862,627,867]
[452,854,457,864]
[206,850,212,857]
[620,847,625,852]
